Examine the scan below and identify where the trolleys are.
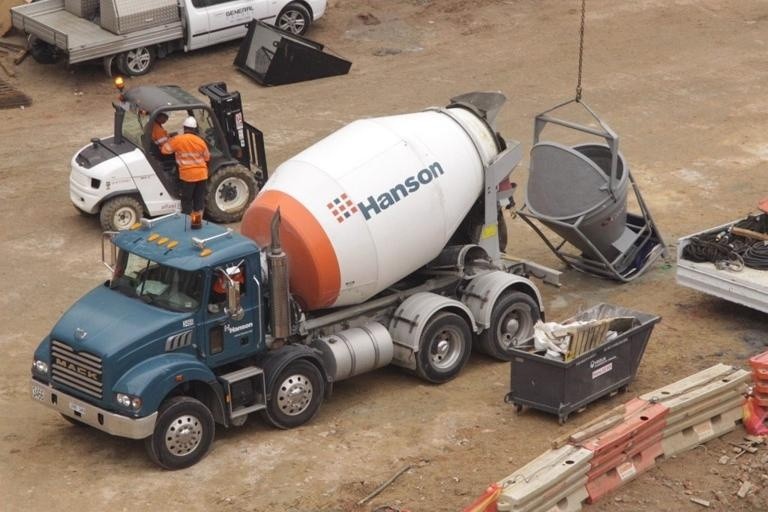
[502,301,663,426]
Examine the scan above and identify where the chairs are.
[141,135,175,161]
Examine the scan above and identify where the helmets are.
[183,116,197,128]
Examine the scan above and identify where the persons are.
[210,265,245,304]
[145,111,178,157]
[160,115,210,218]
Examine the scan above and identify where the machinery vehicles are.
[67,73,271,239]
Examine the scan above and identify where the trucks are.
[29,89,564,474]
[8,0,330,78]
